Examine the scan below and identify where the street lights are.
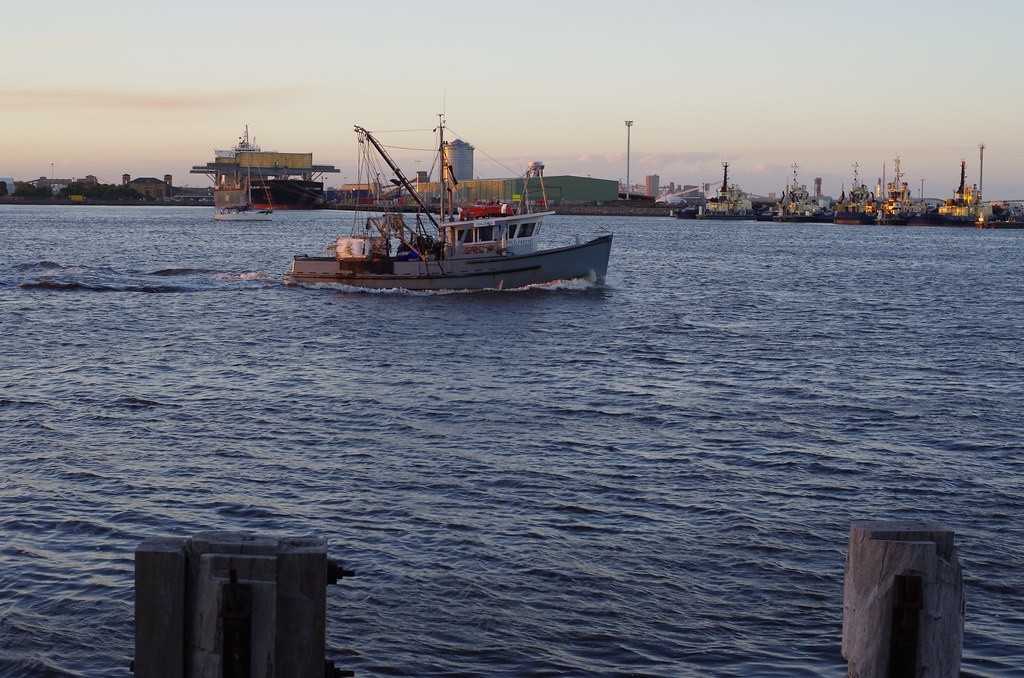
[625,121,633,199]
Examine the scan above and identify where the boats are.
[289,88,614,292]
[214,202,274,221]
[696,155,1024,229]
